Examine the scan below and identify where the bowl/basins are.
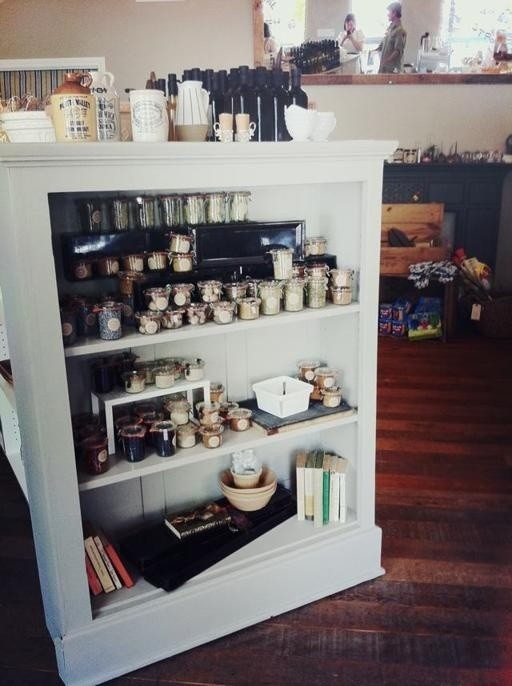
[217,468,277,511]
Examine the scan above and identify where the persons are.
[368,2,406,74]
[262,23,276,54]
[495,33,508,56]
[338,14,366,53]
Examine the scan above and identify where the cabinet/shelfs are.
[324,55,360,74]
[381,160,507,297]
[0,134,401,686]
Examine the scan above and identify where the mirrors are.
[253,0,512,86]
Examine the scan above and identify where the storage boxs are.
[380,203,450,276]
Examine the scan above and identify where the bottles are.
[271,69,293,140]
[229,192,250,222]
[224,68,239,141]
[233,66,256,141]
[98,303,122,340]
[288,69,307,109]
[182,75,184,81]
[200,71,205,89]
[192,68,200,80]
[123,255,142,271]
[184,70,191,80]
[80,198,100,233]
[394,148,402,160]
[109,198,129,231]
[305,239,325,255]
[169,235,191,254]
[283,72,289,91]
[206,69,216,141]
[248,69,256,88]
[185,194,205,225]
[266,70,273,88]
[167,74,178,141]
[216,70,230,141]
[159,194,183,227]
[50,73,97,142]
[153,81,157,89]
[206,192,227,225]
[290,39,342,74]
[98,257,119,276]
[90,72,121,142]
[213,72,220,92]
[270,54,275,67]
[404,150,416,163]
[158,79,165,95]
[173,255,193,271]
[253,66,274,141]
[147,253,167,270]
[136,196,154,231]
[75,260,91,278]
